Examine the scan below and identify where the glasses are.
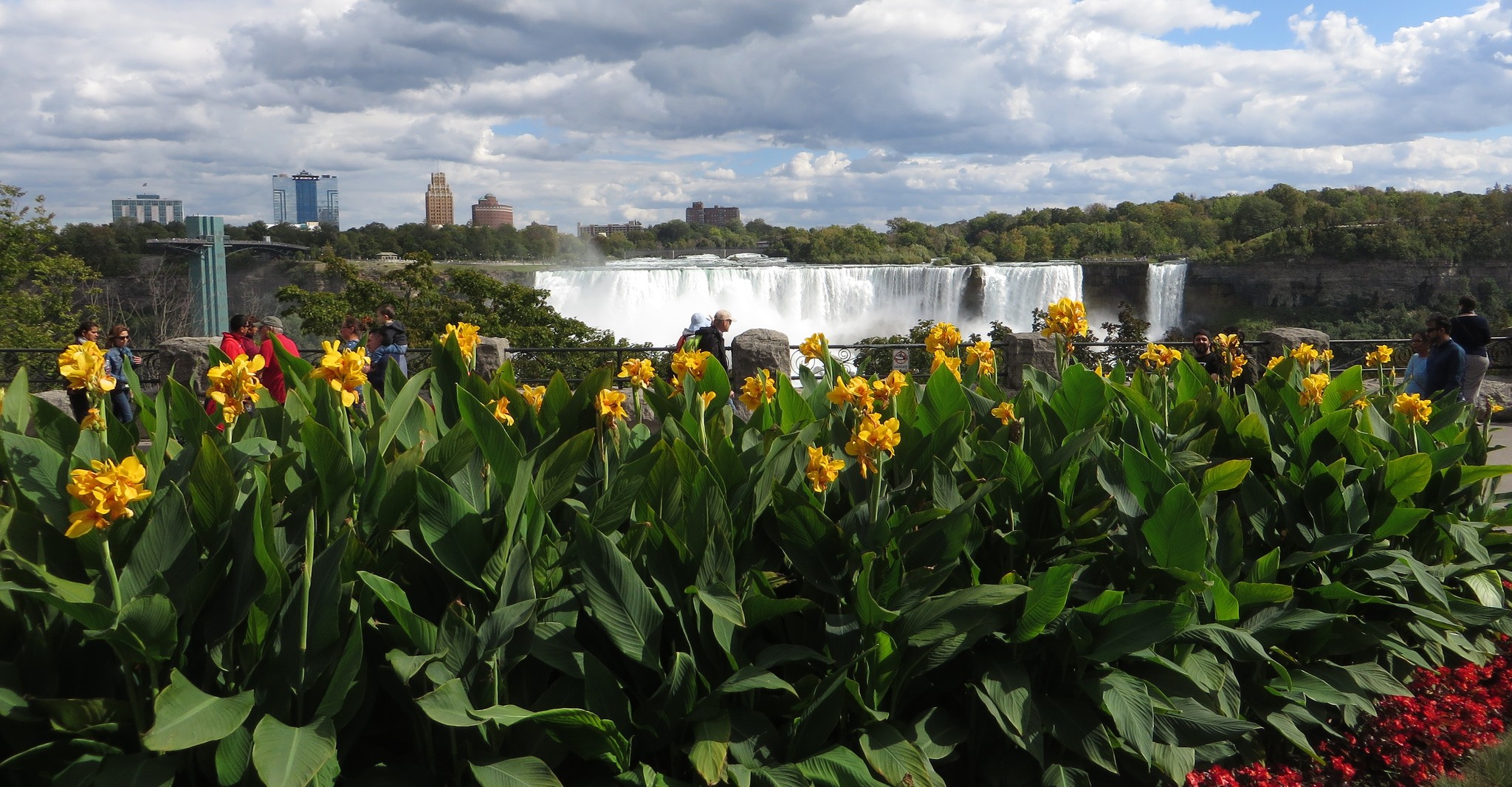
[115,335,130,340]
[1426,328,1440,334]
[725,320,732,325]
[1411,338,1423,343]
[245,324,250,327]
[339,326,350,328]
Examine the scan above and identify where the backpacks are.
[676,334,695,353]
[682,335,703,353]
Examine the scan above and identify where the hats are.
[1193,329,1211,341]
[253,316,283,329]
[714,309,737,322]
[689,313,712,330]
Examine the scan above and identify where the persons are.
[253,316,300,408]
[1449,294,1492,410]
[103,323,143,424]
[694,309,737,414]
[1216,325,1259,395]
[340,317,367,419]
[220,314,254,405]
[361,328,408,400]
[241,315,262,392]
[61,320,105,425]
[1425,311,1466,404]
[1403,329,1431,401]
[676,313,712,397]
[370,303,409,369]
[1193,329,1220,385]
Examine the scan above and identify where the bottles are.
[103,359,109,373]
